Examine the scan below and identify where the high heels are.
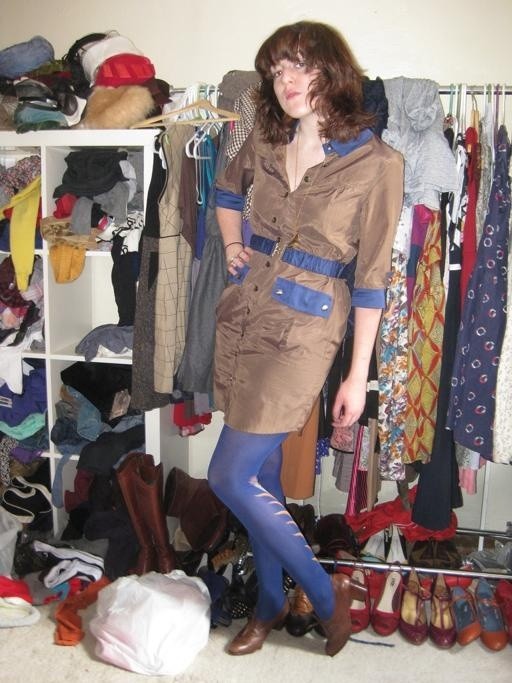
[320,572,368,658]
[227,597,290,655]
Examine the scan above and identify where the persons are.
[201,17,410,660]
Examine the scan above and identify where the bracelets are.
[224,240,245,247]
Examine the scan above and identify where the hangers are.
[127,83,244,161]
[444,81,508,154]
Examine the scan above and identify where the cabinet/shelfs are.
[0,127,189,539]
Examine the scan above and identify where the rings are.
[231,256,237,263]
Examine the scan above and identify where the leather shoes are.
[286,549,508,637]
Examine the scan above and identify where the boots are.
[135,461,176,574]
[117,451,155,576]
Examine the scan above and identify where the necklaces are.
[291,123,328,190]
